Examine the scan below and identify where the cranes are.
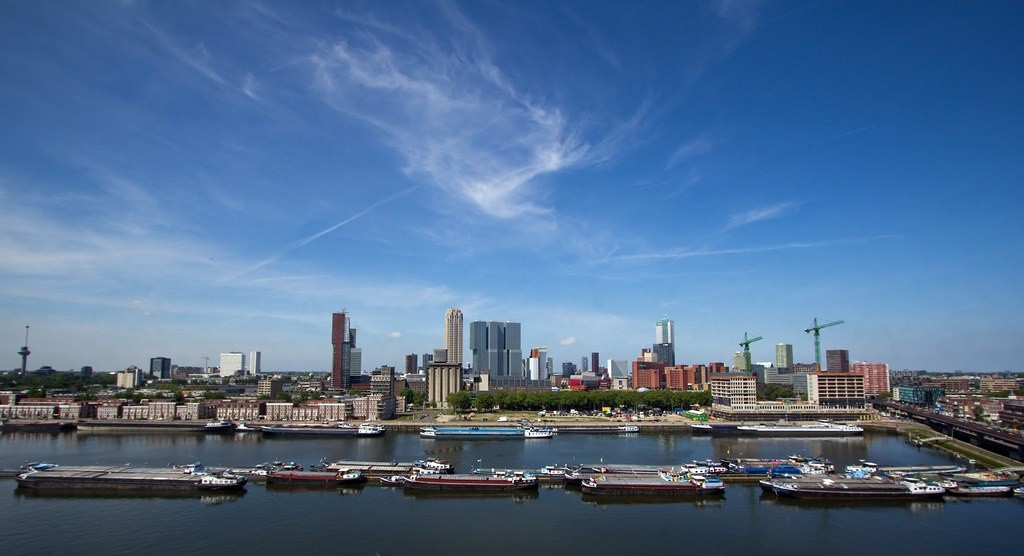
[804,317,845,372]
[738,332,764,375]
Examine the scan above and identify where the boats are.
[205,419,236,430]
[0,453,1024,511]
[234,423,387,434]
[690,417,864,437]
[418,426,640,438]
